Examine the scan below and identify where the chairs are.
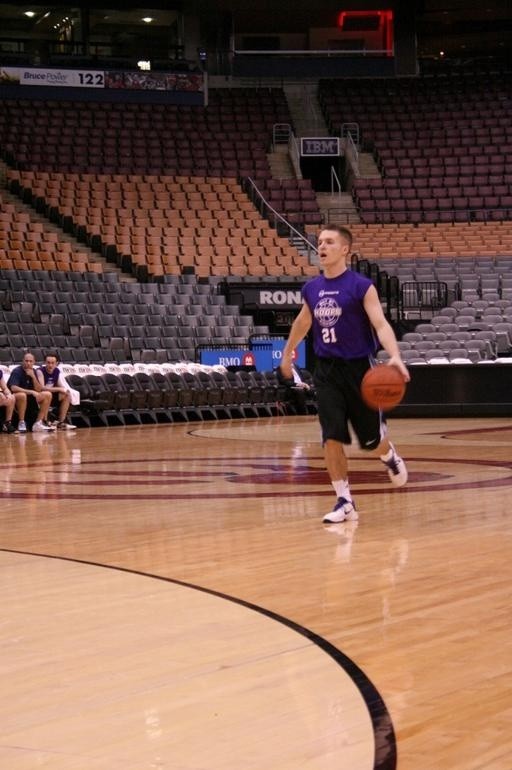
[0,72,512,430]
[381,441,408,489]
[323,497,359,522]
[3,420,77,432]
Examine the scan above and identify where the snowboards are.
[362,365,405,409]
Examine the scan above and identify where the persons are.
[323,524,411,614]
[276,222,413,524]
[0,351,77,435]
